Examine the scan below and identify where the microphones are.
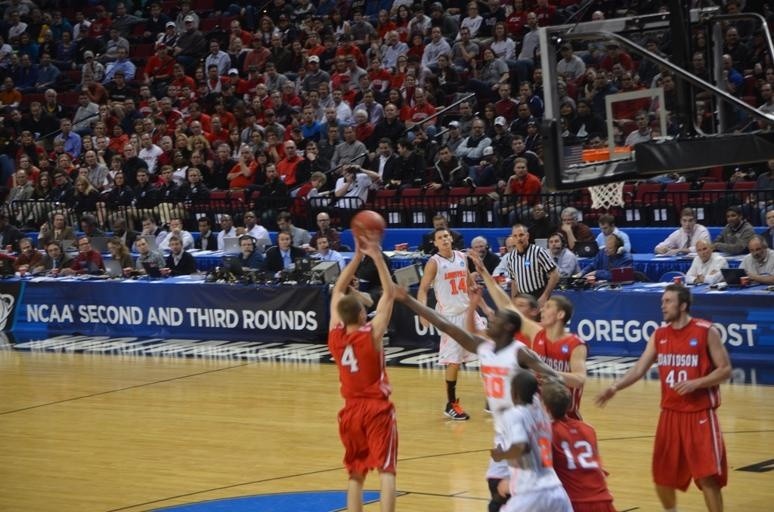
[553,247,561,249]
[326,246,331,249]
[287,245,292,248]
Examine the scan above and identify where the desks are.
[1,242,774,375]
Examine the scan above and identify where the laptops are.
[720,268,747,286]
[575,241,599,257]
[423,232,438,254]
[611,267,635,285]
[60,235,270,278]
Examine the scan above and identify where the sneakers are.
[443,398,469,420]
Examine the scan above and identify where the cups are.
[122,268,130,278]
[51,269,58,278]
[6,245,11,253]
[19,267,26,277]
[672,276,681,285]
[499,246,506,256]
[739,276,751,286]
[394,242,408,252]
[587,275,595,288]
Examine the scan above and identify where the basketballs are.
[351,210,386,239]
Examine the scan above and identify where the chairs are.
[2,1,774,241]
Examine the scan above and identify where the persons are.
[0,2,773,291]
[390,223,614,509]
[326,230,399,512]
[596,282,733,512]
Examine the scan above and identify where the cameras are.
[346,166,356,180]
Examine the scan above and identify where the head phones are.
[546,232,565,248]
[315,236,331,250]
[275,231,293,246]
[503,238,513,250]
[485,244,490,250]
[610,232,625,254]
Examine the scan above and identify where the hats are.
[227,68,239,75]
[493,116,507,126]
[307,54,320,64]
[165,21,176,29]
[157,43,166,50]
[447,120,461,129]
[480,146,497,159]
[182,16,194,24]
